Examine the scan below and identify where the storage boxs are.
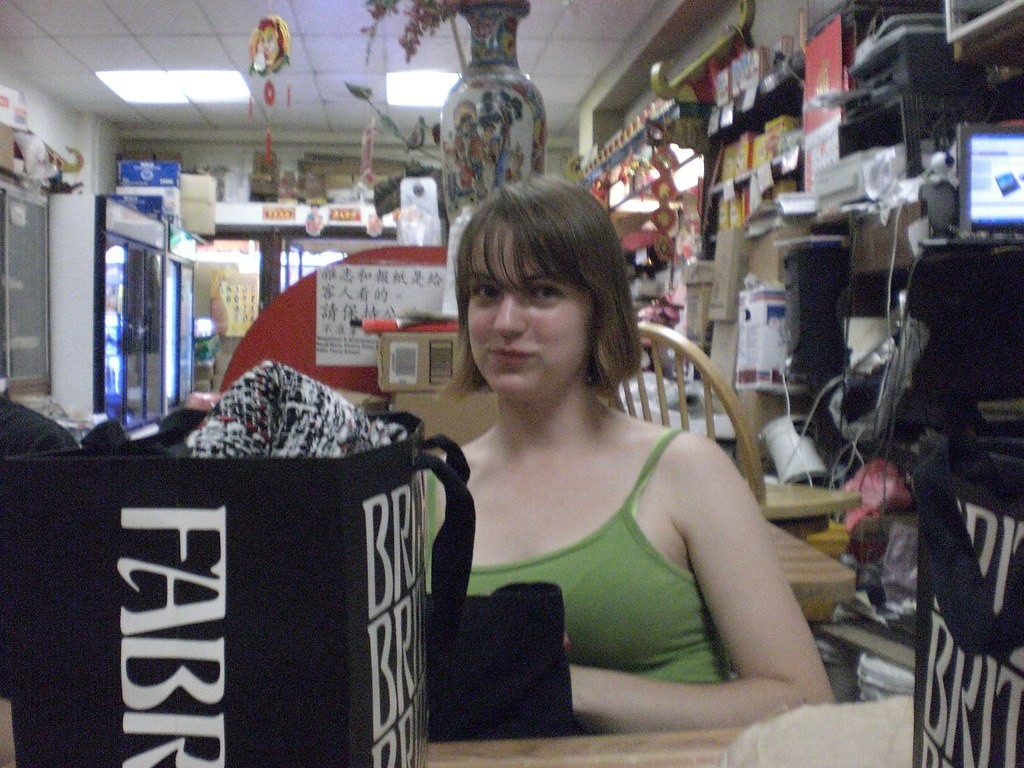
[390,392,501,456]
[180,174,216,237]
[377,332,466,392]
[0,84,28,132]
[739,46,770,92]
[155,151,181,166]
[769,34,794,72]
[117,159,180,188]
[717,66,733,107]
[684,114,951,477]
[325,175,354,203]
[731,55,742,97]
[353,175,390,190]
[116,186,182,227]
[0,123,15,171]
[121,150,155,161]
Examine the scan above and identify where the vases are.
[439,0,546,240]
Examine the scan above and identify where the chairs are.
[600,322,769,505]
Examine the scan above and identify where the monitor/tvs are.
[954,120,1024,242]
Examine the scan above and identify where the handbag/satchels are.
[0,394,478,768]
[884,245,1024,658]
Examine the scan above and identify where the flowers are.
[361,0,487,65]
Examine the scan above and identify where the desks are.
[764,520,857,605]
[762,480,863,518]
[424,726,760,768]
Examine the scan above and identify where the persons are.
[0,379,77,457]
[420,175,837,735]
